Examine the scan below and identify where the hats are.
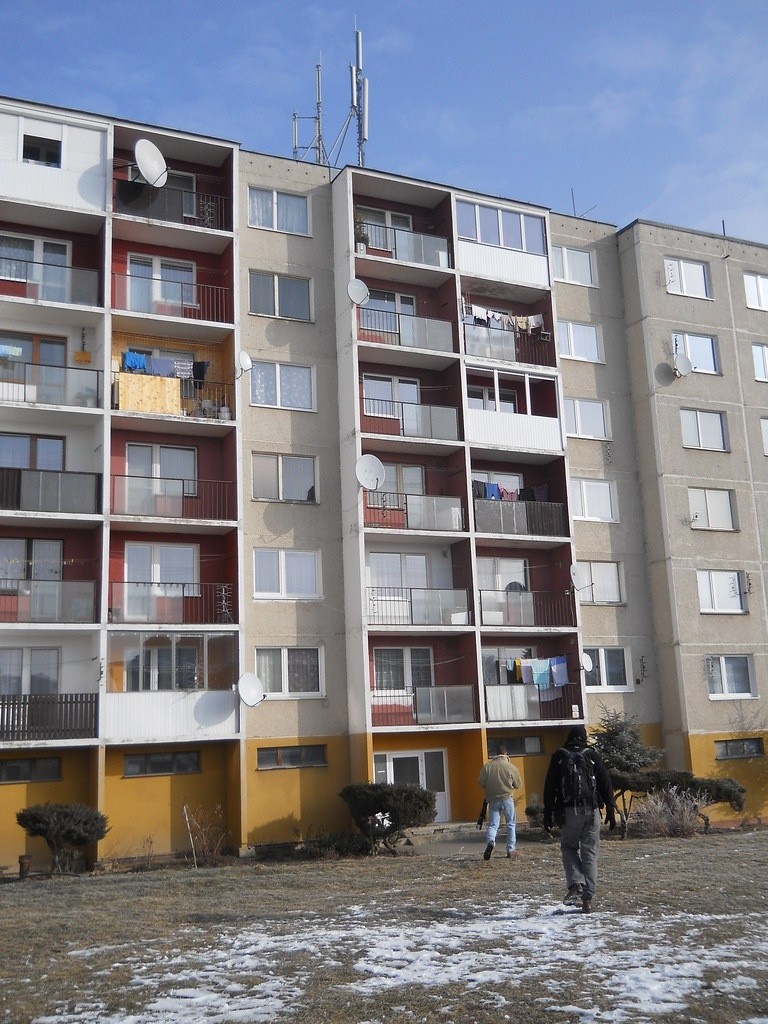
[568,726,586,740]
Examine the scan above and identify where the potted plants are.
[354,202,369,254]
[75,387,97,407]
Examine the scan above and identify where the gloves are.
[604,806,616,831]
[542,809,553,833]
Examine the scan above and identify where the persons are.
[478,744,522,860]
[542,726,616,912]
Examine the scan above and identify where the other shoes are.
[507,852,514,858]
[581,898,591,914]
[484,842,493,860]
[563,883,583,906]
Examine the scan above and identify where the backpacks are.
[557,747,598,807]
